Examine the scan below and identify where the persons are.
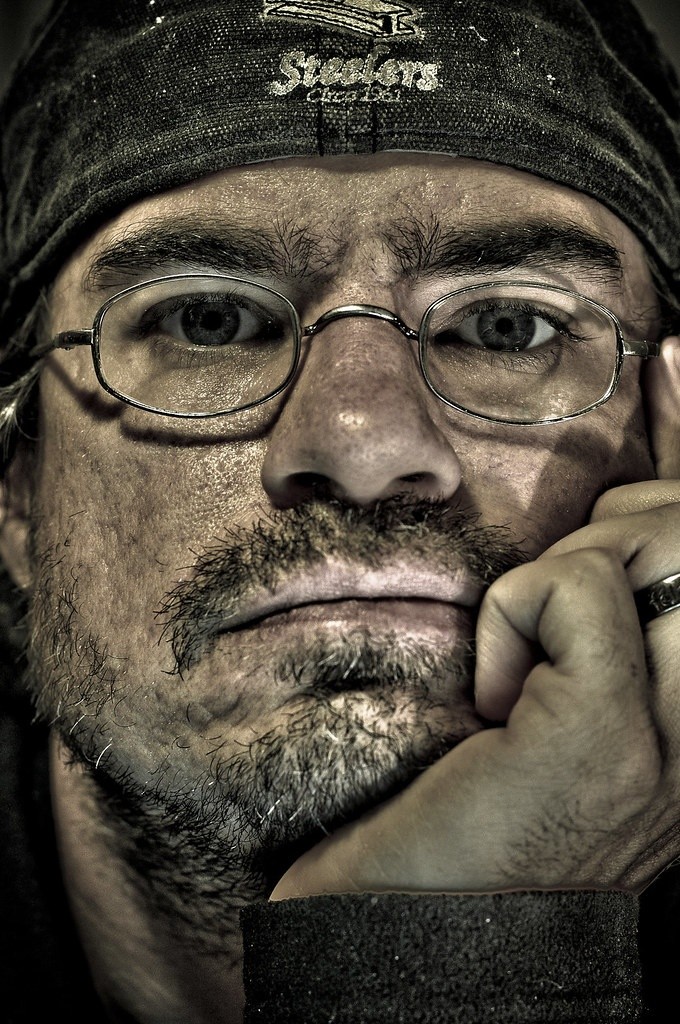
[0,0,680,1024]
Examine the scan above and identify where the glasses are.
[29,273,661,426]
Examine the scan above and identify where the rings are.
[633,572,680,626]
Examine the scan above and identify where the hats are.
[0,0,680,315]
